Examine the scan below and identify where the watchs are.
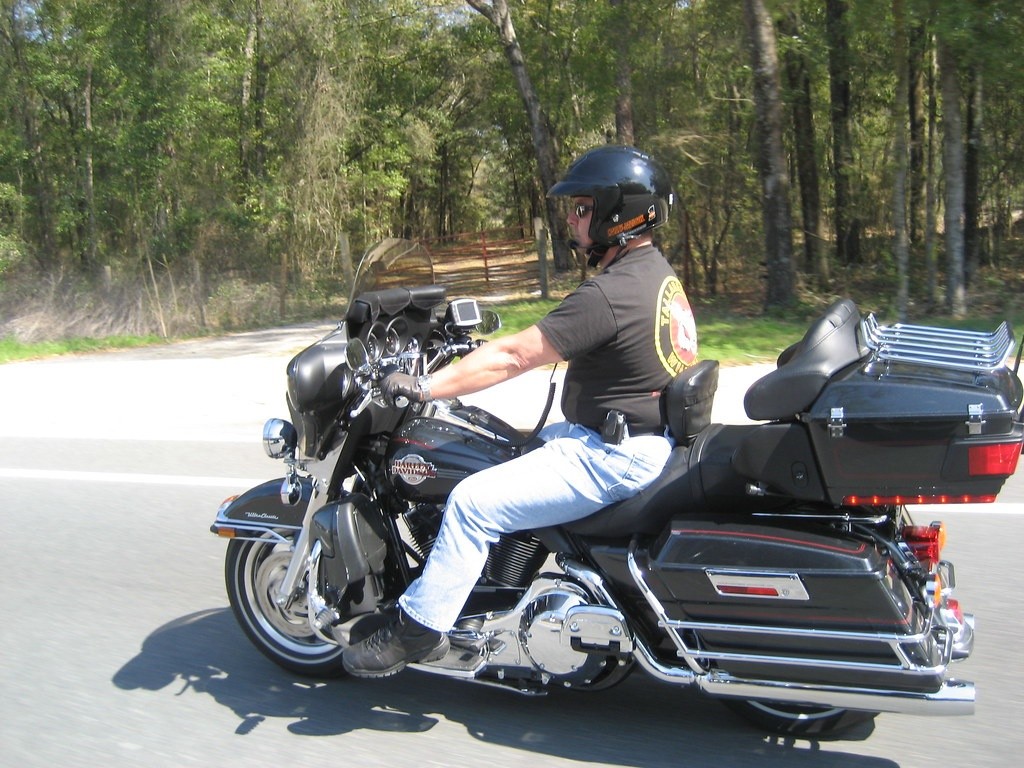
[418,373,433,402]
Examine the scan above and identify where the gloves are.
[379,371,421,411]
[476,339,488,347]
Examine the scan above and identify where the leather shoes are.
[342,608,451,679]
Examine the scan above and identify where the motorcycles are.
[210,238,1024,738]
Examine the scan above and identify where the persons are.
[344,145,698,678]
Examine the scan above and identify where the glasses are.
[575,203,593,219]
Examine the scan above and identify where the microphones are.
[568,240,600,250]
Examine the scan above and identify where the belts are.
[598,422,664,436]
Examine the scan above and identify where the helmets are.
[546,145,674,244]
